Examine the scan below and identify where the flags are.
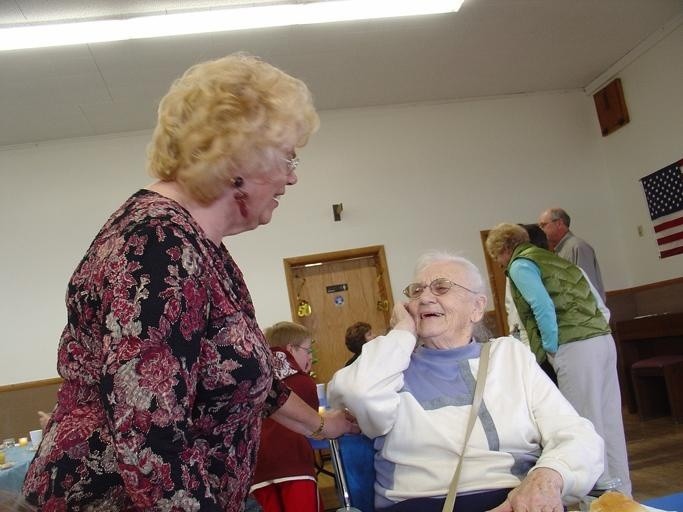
[638,155,683,259]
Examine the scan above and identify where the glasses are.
[272,151,303,178]
[402,277,483,300]
[292,343,313,355]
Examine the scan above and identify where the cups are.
[0,452,5,465]
[316,383,325,398]
[18,437,28,448]
[2,437,15,448]
[28,429,42,448]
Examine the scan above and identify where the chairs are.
[249,475,322,512]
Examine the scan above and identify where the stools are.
[630,354,683,421]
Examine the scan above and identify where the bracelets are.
[307,414,325,439]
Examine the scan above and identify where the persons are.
[20,52,363,512]
[485,210,633,499]
[247,322,320,512]
[343,321,375,367]
[326,249,605,512]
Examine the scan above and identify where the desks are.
[0,441,40,511]
[616,311,681,414]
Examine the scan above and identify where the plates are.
[0,462,13,469]
[25,446,38,451]
